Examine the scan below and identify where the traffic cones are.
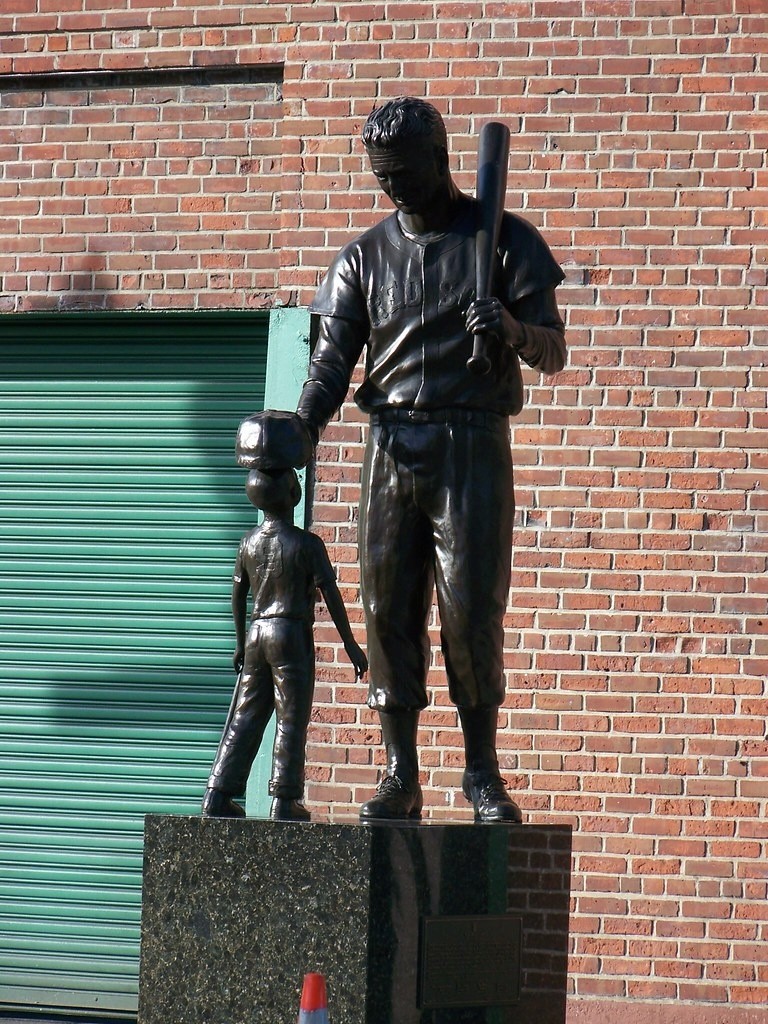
[292,973,329,1024]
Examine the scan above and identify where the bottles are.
[297,974,328,1024]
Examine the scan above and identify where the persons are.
[200,95,569,825]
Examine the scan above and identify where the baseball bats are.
[466,122,513,377]
